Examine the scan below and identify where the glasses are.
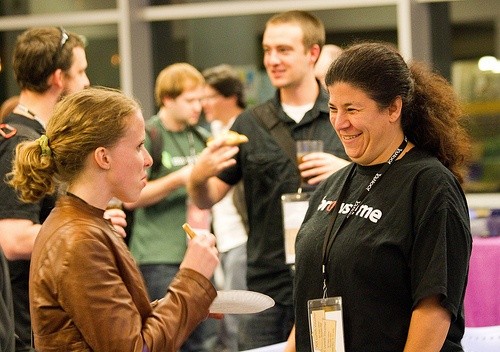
[38,26,69,82]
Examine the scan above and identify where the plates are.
[209,290,276,315]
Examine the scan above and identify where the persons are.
[0,25,90,351]
[186,11,353,352]
[122,62,207,351]
[282,42,473,352]
[201,64,247,352]
[4,86,220,351]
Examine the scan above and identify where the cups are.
[296,140,323,188]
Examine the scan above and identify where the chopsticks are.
[182,223,197,239]
